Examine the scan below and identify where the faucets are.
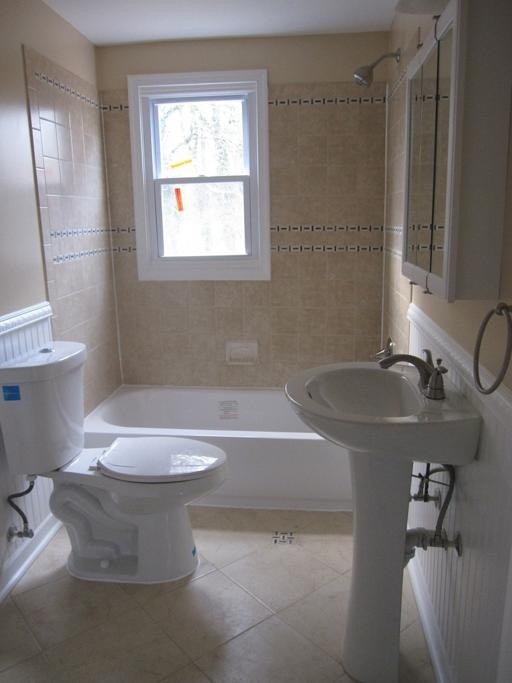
[379,353,437,389]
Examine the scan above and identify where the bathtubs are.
[83,385,354,501]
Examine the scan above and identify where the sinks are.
[284,362,483,465]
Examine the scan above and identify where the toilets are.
[0,342,226,584]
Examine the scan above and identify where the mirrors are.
[400,2,510,306]
